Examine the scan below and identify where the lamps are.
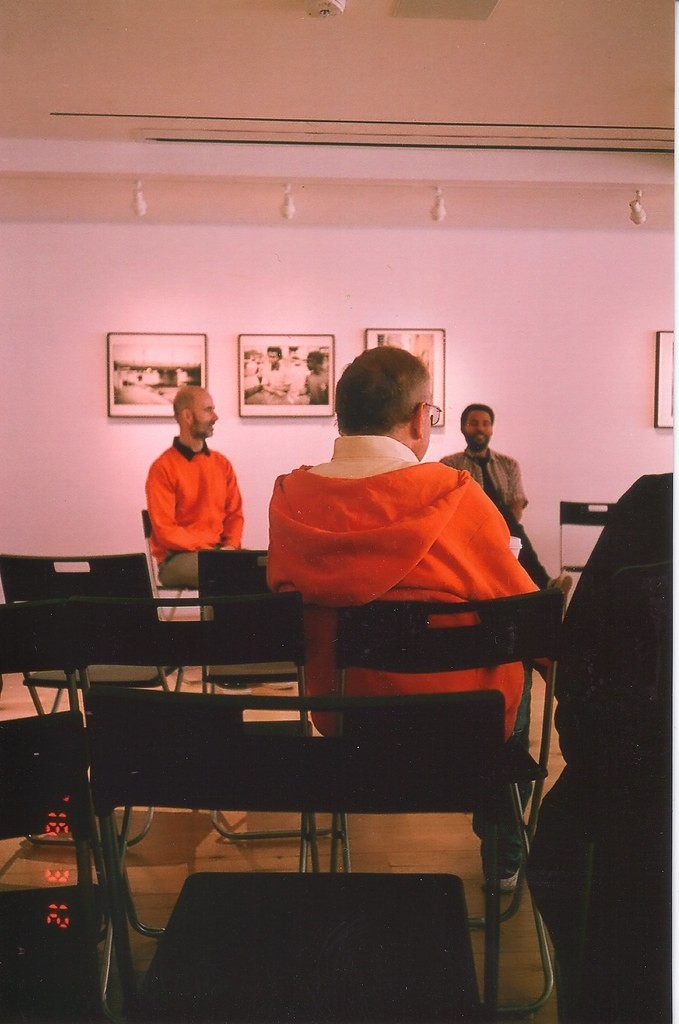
[430,186,446,220]
[130,181,146,218]
[281,184,296,221]
[627,190,647,225]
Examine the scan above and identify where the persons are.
[524,470,671,1023]
[145,385,244,590]
[113,366,191,397]
[241,346,329,405]
[436,404,571,618]
[265,347,545,892]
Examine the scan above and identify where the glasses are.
[426,402,442,426]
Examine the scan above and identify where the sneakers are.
[262,682,294,690]
[547,571,573,608]
[213,681,252,696]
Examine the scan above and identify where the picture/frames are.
[238,333,336,417]
[365,327,446,428]
[107,332,207,417]
[653,331,674,429]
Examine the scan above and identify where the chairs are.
[0,500,673,1024]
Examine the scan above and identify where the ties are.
[479,458,518,530]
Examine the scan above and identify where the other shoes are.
[479,866,520,894]
